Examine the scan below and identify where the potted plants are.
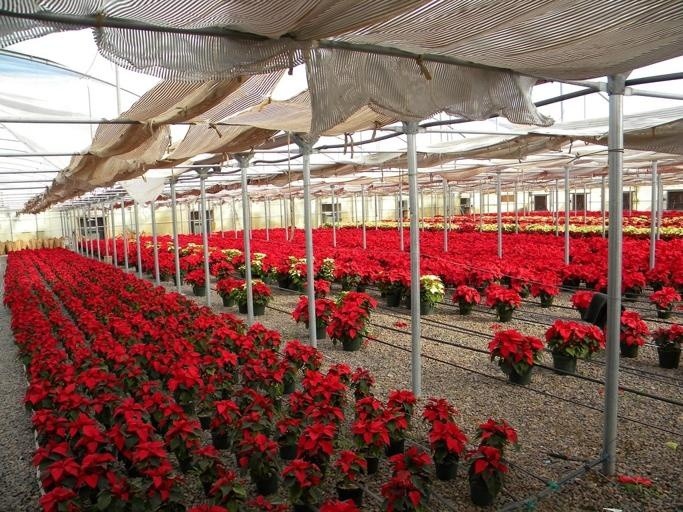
[1,208,519,510]
[647,287,681,316]
[519,209,683,303]
[544,319,605,374]
[487,330,543,386]
[570,291,607,319]
[650,325,682,368]
[619,310,647,357]
